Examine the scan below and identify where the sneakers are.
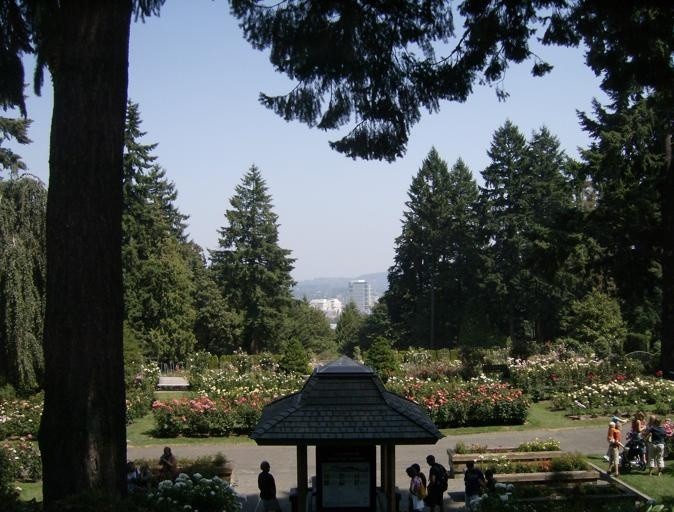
[624,447,630,451]
[608,470,612,477]
[616,472,621,479]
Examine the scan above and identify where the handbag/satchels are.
[418,483,426,499]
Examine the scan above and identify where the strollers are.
[620,437,646,474]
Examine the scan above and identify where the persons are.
[411,463,426,501]
[139,462,152,479]
[258,460,281,511]
[477,469,496,496]
[463,460,483,511]
[157,446,176,479]
[127,461,140,479]
[425,454,448,512]
[606,407,674,477]
[405,466,425,512]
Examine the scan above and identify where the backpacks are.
[433,464,448,491]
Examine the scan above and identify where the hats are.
[612,415,618,421]
[616,422,626,427]
